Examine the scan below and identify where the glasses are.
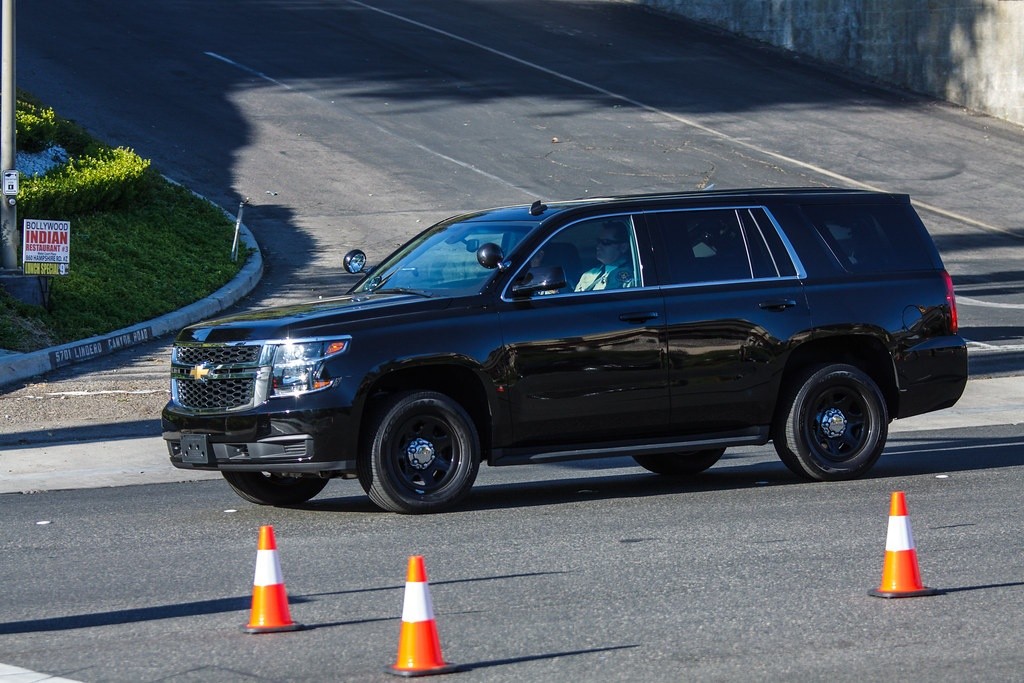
[596,237,625,246]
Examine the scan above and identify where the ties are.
[584,266,605,292]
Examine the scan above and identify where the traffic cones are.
[385,555,458,678]
[239,526,305,635]
[867,491,944,598]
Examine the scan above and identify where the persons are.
[530,227,635,291]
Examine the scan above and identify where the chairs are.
[544,244,574,293]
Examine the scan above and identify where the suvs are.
[160,188,969,518]
